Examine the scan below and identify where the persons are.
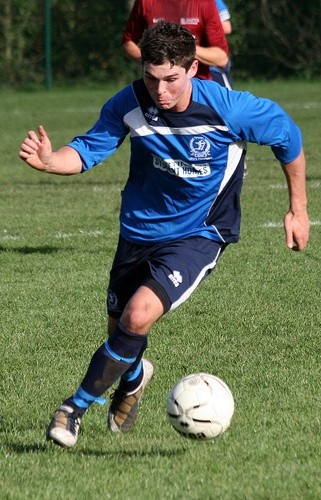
[119,0,234,91]
[18,20,312,451]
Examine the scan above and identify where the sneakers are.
[46,397,86,450]
[107,359,154,435]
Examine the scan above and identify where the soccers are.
[169,374,238,439]
